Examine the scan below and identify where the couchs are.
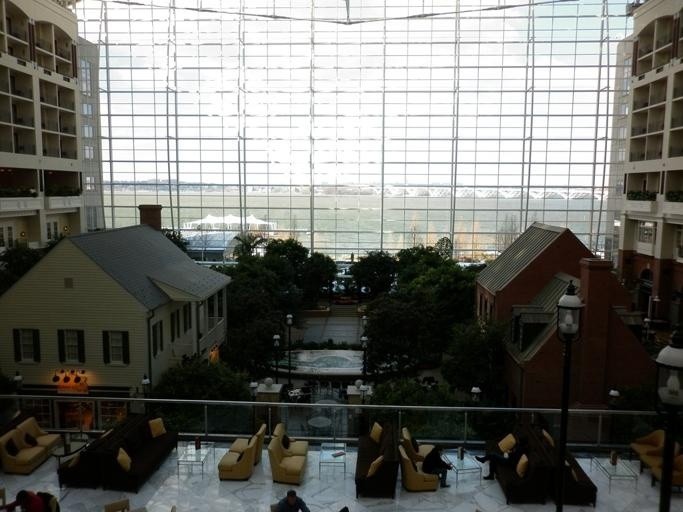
[485,419,597,507]
[355,414,398,499]
[56,411,177,494]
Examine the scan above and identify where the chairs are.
[627,430,683,495]
[270,503,280,512]
[398,426,438,492]
[0,417,60,512]
[104,499,176,512]
[218,423,311,484]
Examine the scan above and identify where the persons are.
[0,489,46,511]
[297,384,312,409]
[275,489,311,512]
[473,427,520,480]
[421,443,452,487]
[279,384,294,403]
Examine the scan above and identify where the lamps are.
[52,367,85,383]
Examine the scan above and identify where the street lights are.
[604,384,623,442]
[360,384,369,417]
[13,369,27,417]
[649,293,661,331]
[141,374,152,416]
[469,384,483,432]
[360,334,369,386]
[548,275,589,511]
[361,313,370,332]
[285,313,296,386]
[642,315,653,345]
[643,324,683,512]
[270,333,283,385]
[248,381,260,434]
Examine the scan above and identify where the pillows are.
[366,455,384,478]
[370,422,383,443]
